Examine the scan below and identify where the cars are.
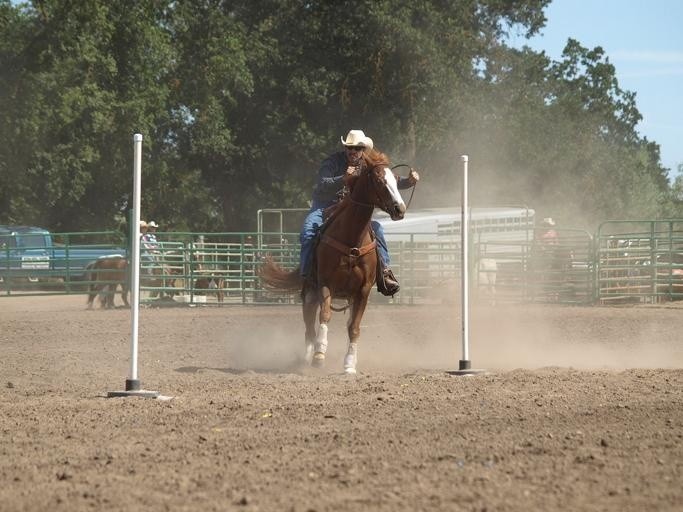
[504,229,683,305]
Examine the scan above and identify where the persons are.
[299,129,419,307]
[143,218,159,254]
[139,221,157,281]
[534,217,558,252]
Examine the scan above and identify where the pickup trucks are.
[0,224,125,293]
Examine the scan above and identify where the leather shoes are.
[301,280,318,305]
[379,272,400,292]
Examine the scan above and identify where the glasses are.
[345,145,363,151]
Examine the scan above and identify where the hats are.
[340,128,374,150]
[139,220,159,228]
[538,217,555,226]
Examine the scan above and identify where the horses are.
[85,243,225,310]
[256,147,406,374]
